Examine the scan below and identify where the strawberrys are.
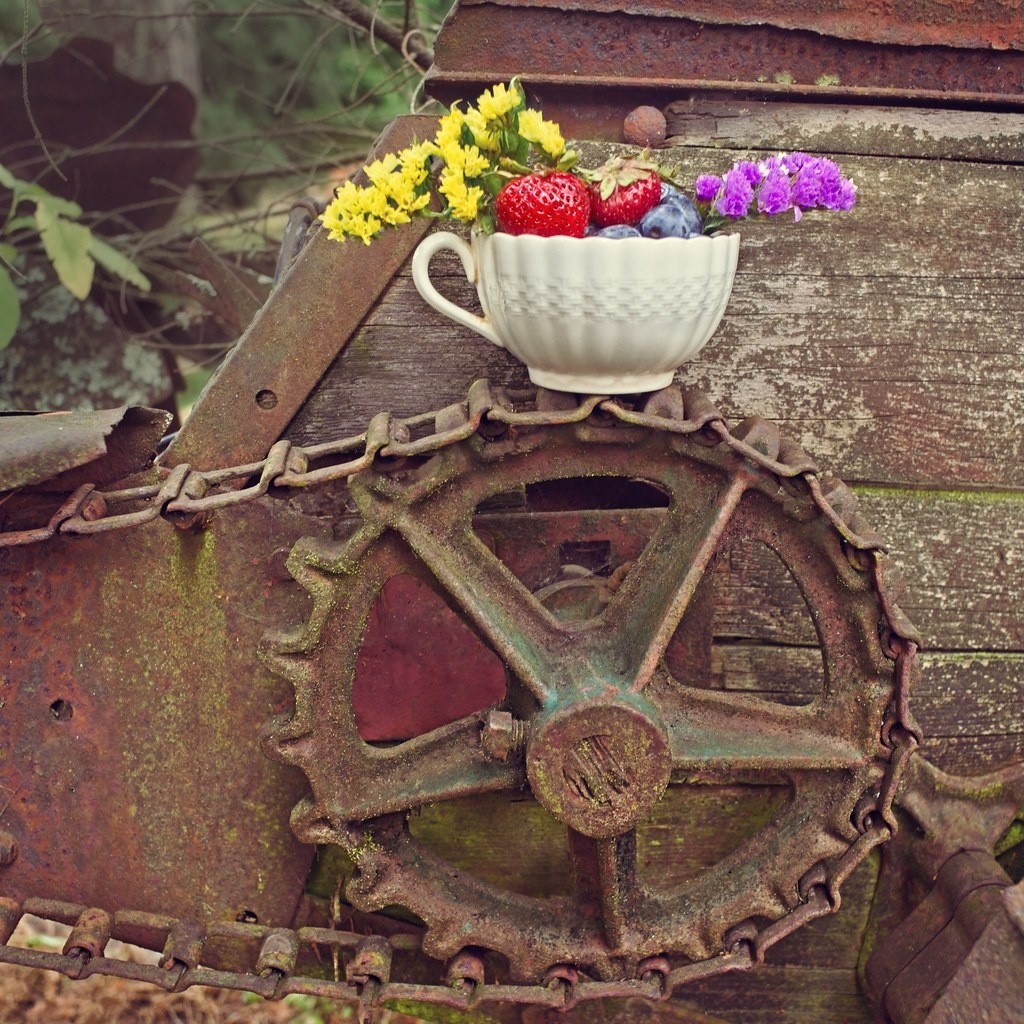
[587,157,661,226]
[495,166,590,240]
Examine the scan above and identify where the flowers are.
[316,74,868,245]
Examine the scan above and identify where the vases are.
[408,228,740,395]
